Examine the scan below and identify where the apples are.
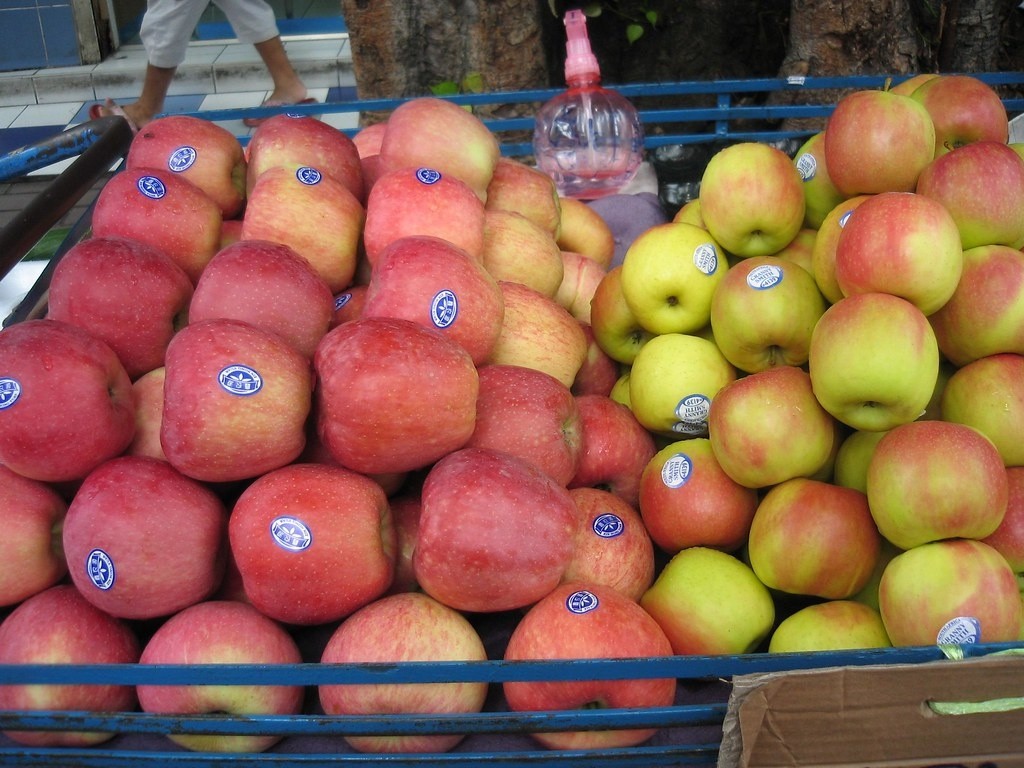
[590,72,1024,652]
[1,96,678,747]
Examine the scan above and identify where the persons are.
[88,0,318,147]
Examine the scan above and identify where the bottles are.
[531,7,643,197]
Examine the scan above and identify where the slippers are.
[243,96,318,126]
[88,101,142,139]
